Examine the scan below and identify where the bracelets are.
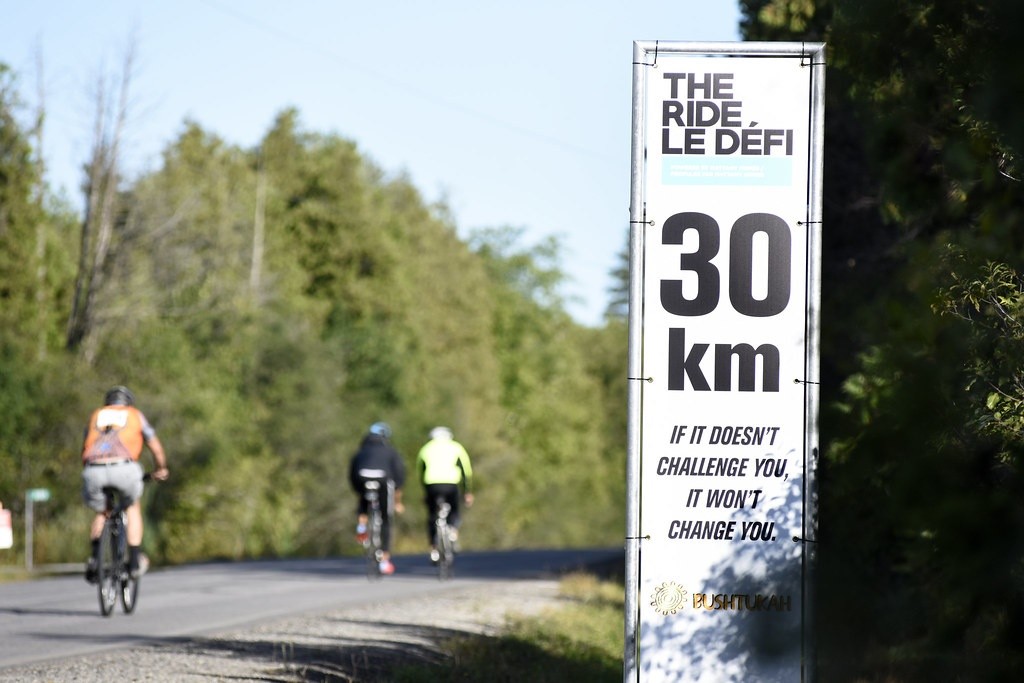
[155,465,168,469]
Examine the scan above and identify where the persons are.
[415,426,475,566]
[347,423,409,575]
[81,385,170,586]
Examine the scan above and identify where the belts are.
[87,459,132,467]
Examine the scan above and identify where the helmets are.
[370,422,392,438]
[104,385,134,404]
[430,426,453,439]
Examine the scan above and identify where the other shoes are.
[85,557,97,584]
[129,553,150,578]
[355,524,369,545]
[379,561,396,574]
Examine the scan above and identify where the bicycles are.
[90,472,154,615]
[362,484,401,578]
[434,500,456,580]
